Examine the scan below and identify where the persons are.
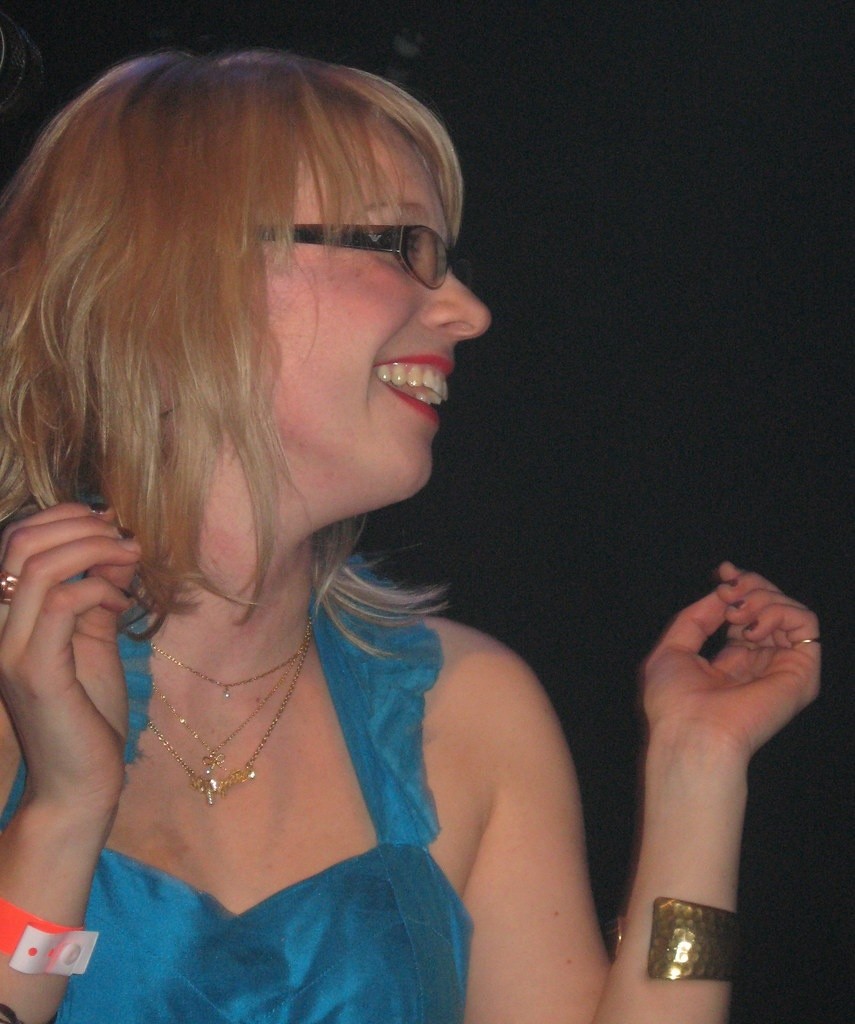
[0,46,822,1024]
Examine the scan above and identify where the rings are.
[792,638,821,646]
[0,572,19,604]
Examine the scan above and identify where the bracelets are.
[615,894,746,983]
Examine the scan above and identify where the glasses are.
[257,222,466,291]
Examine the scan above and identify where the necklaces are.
[149,612,314,807]
